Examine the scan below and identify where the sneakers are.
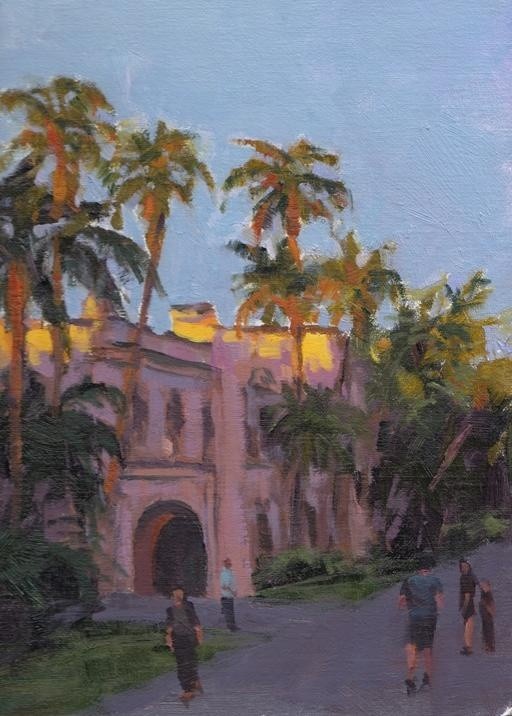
[179,677,204,701]
[404,674,432,692]
[460,646,496,656]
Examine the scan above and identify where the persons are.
[165,585,205,704]
[474,577,495,652]
[456,557,479,656]
[397,558,445,694]
[218,555,239,635]
